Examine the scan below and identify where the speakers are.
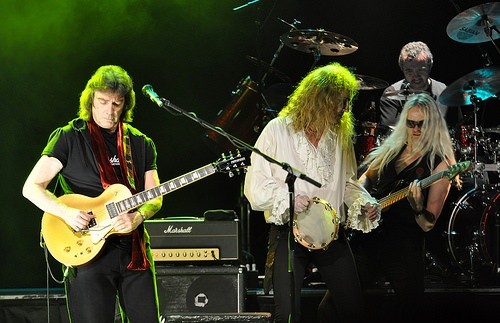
[155,265,245,313]
[0,295,74,323]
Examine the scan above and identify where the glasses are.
[407,120,424,129]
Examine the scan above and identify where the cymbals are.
[438,65,500,106]
[446,2,500,43]
[353,73,389,89]
[292,196,341,252]
[280,30,358,55]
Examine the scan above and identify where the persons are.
[379,40,450,135]
[316,93,462,323]
[244,62,381,323]
[22,65,163,323]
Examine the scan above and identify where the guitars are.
[41,150,251,268]
[345,158,473,245]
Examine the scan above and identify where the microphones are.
[141,85,164,107]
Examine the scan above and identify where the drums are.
[446,184,500,285]
[293,199,339,249]
[353,121,394,153]
[447,124,477,185]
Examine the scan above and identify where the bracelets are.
[415,208,424,218]
[138,208,145,220]
